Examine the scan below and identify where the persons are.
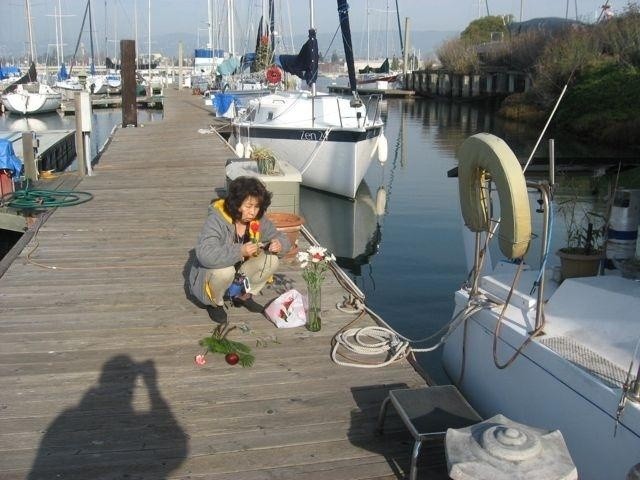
[189,177,291,324]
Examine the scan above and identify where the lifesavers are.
[268,68,281,84]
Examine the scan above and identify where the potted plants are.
[250,143,290,176]
[552,165,615,283]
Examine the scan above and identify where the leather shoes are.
[207,304,228,324]
[233,297,265,313]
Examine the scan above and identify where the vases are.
[263,212,304,247]
[307,284,321,332]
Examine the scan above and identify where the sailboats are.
[437,131,640,479]
[300,180,387,292]
[232,1,388,201]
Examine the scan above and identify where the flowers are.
[195,320,225,366]
[297,243,336,325]
[250,223,261,258]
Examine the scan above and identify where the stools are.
[376,385,483,480]
